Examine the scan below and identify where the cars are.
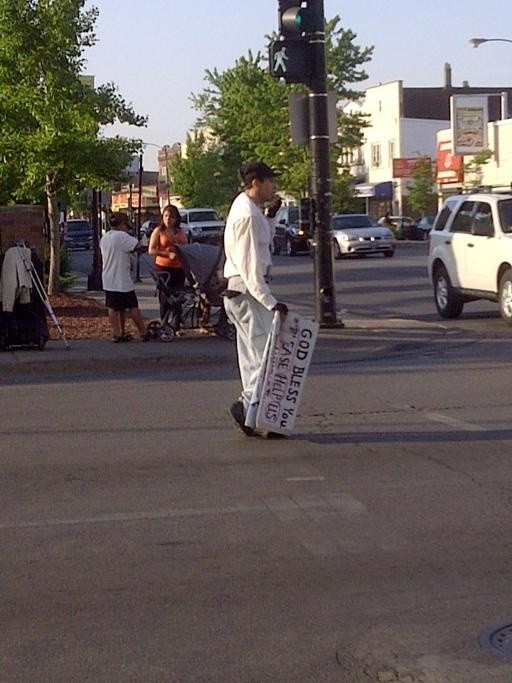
[269,204,435,258]
[140,219,161,237]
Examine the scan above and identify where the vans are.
[60,218,93,249]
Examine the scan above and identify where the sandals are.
[111,331,163,343]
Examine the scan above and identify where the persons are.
[222,162,290,438]
[381,211,397,227]
[97,212,159,341]
[148,204,190,337]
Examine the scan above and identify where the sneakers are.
[225,401,256,437]
[266,431,286,438]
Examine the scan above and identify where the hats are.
[237,162,283,190]
[109,212,133,230]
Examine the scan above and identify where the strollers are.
[141,240,235,340]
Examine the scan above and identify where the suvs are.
[178,207,225,243]
[424,184,512,324]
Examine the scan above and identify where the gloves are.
[267,201,282,217]
[272,303,288,316]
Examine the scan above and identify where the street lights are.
[468,36,511,50]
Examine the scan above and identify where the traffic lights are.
[268,39,309,76]
[277,0,313,38]
